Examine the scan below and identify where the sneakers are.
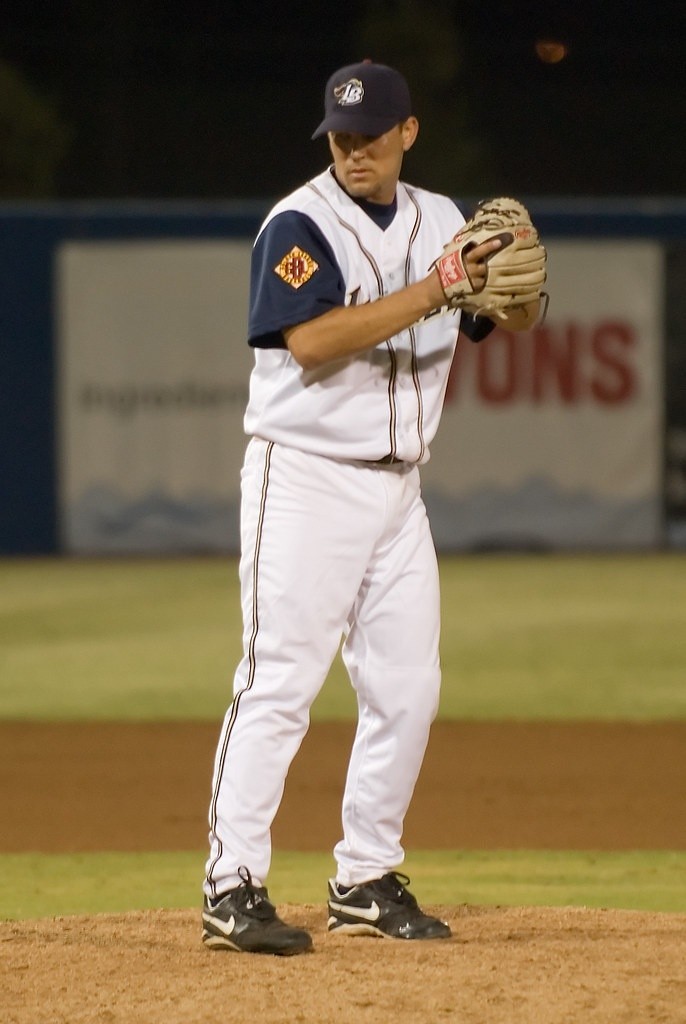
[326,870,453,942]
[200,863,313,957]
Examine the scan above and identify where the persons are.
[204,59,550,956]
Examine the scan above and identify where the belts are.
[367,454,403,464]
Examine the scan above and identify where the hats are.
[311,58,413,139]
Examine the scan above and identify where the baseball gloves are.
[431,195,548,337]
